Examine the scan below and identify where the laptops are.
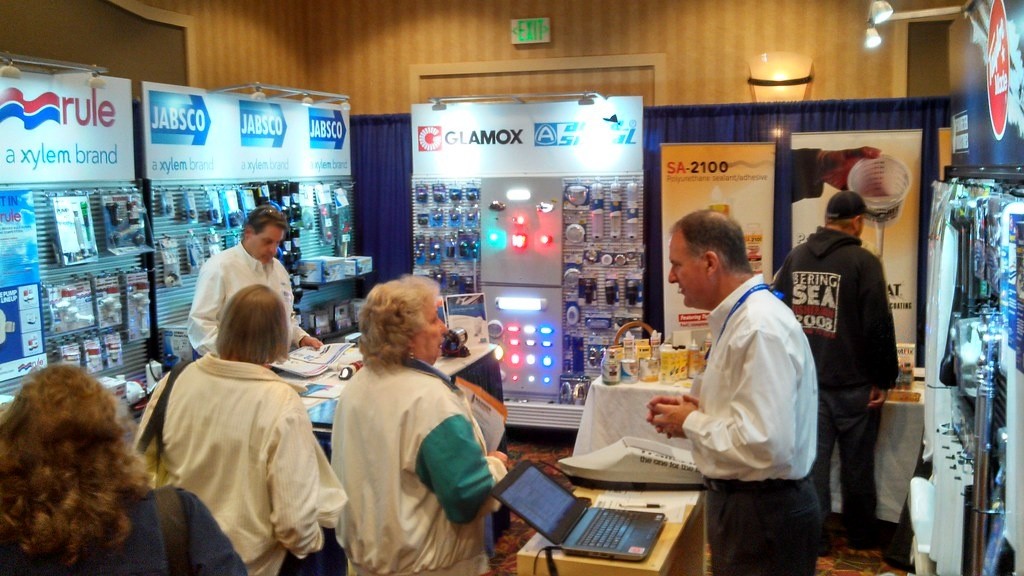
[489,460,666,561]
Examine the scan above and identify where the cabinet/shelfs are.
[300,275,366,340]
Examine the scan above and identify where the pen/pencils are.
[619,503,660,508]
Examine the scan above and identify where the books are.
[260,342,356,377]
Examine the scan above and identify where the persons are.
[646,211,822,576]
[187,205,325,374]
[791,147,888,203]
[331,277,508,576]
[130,285,348,576]
[774,192,899,556]
[0,363,245,576]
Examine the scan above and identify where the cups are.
[848,155,911,229]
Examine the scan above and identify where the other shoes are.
[818,536,834,556]
[848,527,895,549]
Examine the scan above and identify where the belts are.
[703,477,809,491]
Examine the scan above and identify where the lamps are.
[495,297,548,310]
[578,95,595,105]
[250,89,265,100]
[341,99,351,110]
[0,61,21,79]
[432,102,447,110]
[747,52,813,102]
[863,0,962,48]
[301,95,313,106]
[84,73,107,89]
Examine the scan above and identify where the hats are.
[827,190,878,220]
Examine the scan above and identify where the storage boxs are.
[301,255,346,283]
[48,196,98,267]
[161,324,203,367]
[345,254,373,276]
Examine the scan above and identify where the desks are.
[571,381,925,524]
[278,343,511,544]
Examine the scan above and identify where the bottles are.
[620,339,660,383]
